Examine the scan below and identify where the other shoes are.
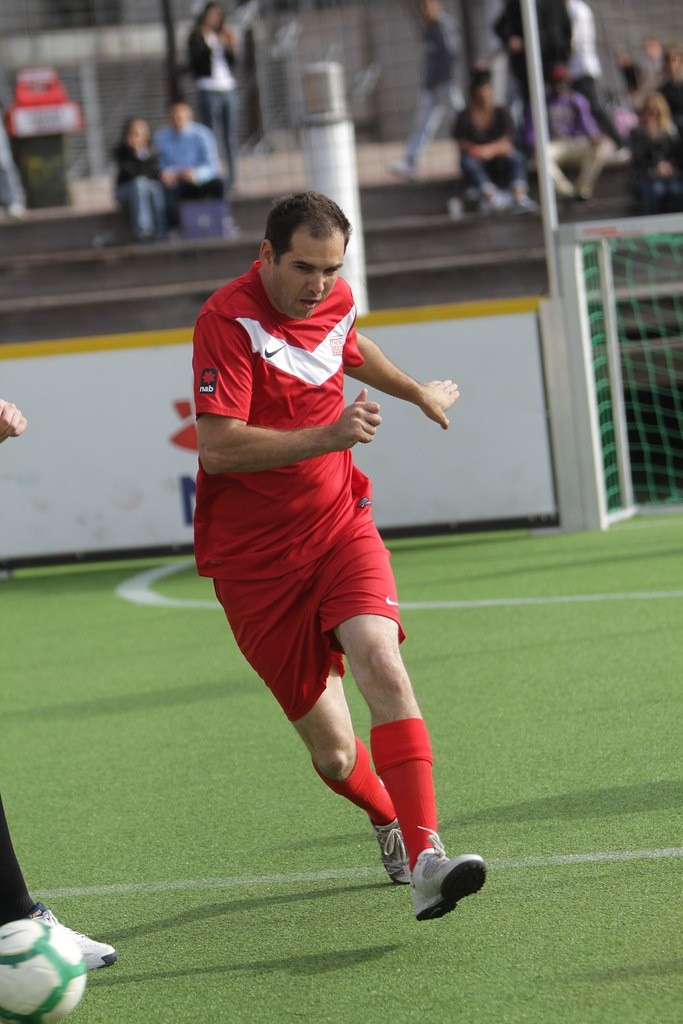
[511,193,537,215]
[576,181,594,199]
[557,181,574,199]
[486,188,508,213]
[388,161,413,184]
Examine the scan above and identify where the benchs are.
[0,150,649,350]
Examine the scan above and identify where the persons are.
[0,398,118,974]
[186,1,245,187]
[390,0,683,218]
[152,102,225,239]
[111,118,171,239]
[193,193,487,924]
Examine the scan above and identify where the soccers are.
[0,918,88,1024]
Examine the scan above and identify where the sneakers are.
[369,816,411,884]
[23,901,117,971]
[410,825,486,921]
